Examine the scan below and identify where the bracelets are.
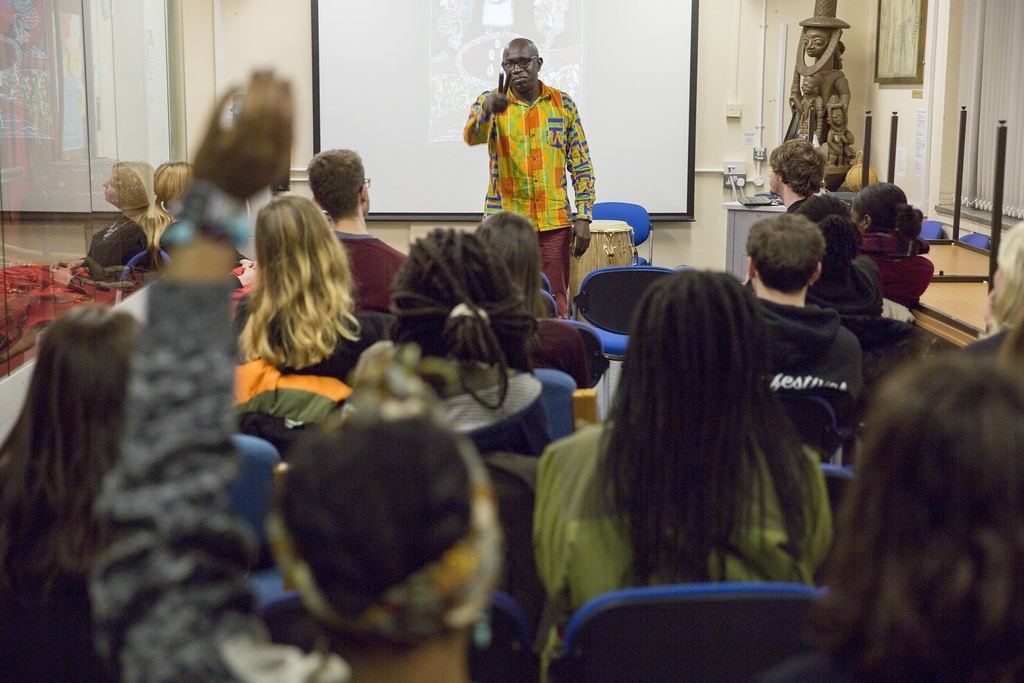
[161,181,251,251]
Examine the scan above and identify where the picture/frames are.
[874,0,928,84]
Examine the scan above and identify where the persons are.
[55,160,154,288]
[464,37,596,317]
[784,0,856,168]
[0,161,1023,682]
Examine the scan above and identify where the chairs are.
[116,203,992,683]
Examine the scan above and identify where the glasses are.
[503,58,537,69]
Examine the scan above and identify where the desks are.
[860,106,1009,347]
